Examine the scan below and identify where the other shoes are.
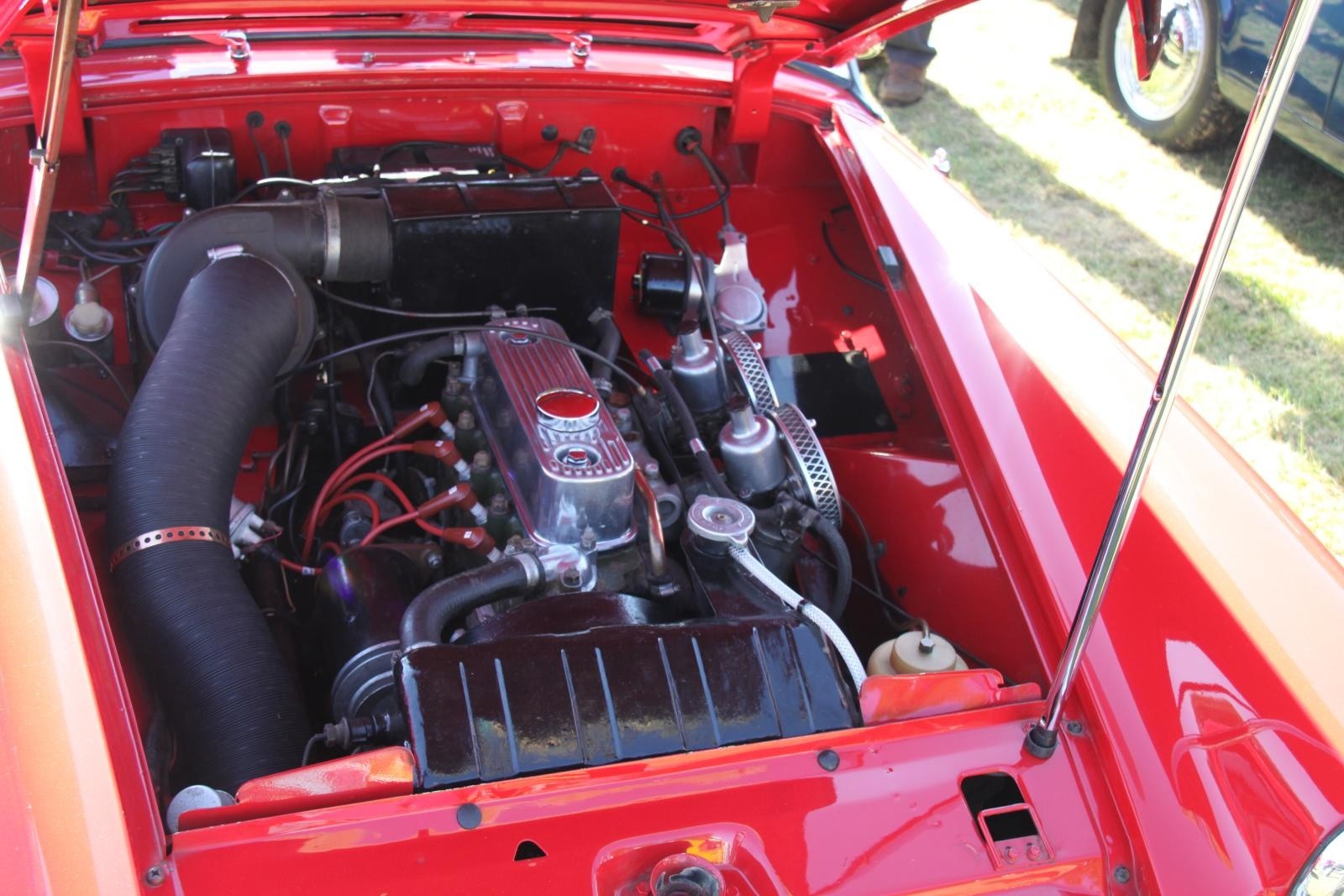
[878,57,926,105]
[856,53,879,71]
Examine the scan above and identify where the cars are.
[1071,1,1344,182]
[1,0,1343,896]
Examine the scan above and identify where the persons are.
[857,19,938,110]
